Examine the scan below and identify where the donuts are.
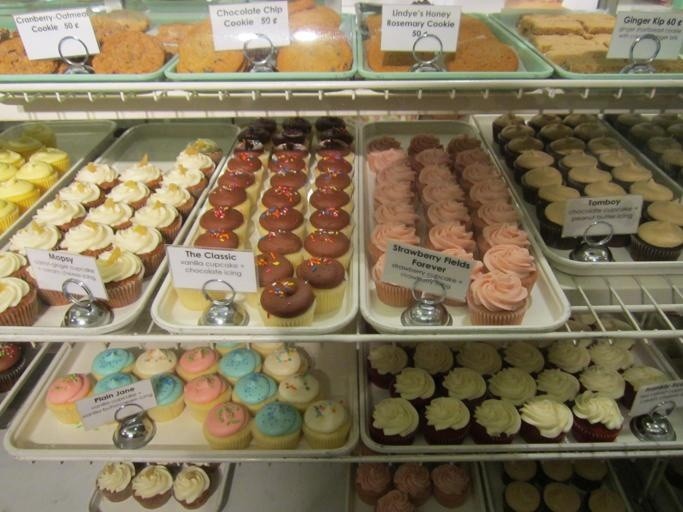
[193,116,356,326]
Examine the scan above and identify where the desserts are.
[356,109,682,512]
[0,123,222,324]
[0,339,352,508]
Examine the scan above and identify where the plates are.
[490,6,683,79]
[346,460,486,511]
[355,12,552,79]
[0,42,180,84]
[87,459,235,512]
[147,119,361,335]
[481,462,631,512]
[164,11,355,80]
[469,112,683,276]
[358,118,571,332]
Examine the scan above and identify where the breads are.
[517,12,683,73]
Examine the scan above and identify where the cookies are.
[1,1,520,77]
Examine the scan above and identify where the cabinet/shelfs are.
[0,78,683,512]
